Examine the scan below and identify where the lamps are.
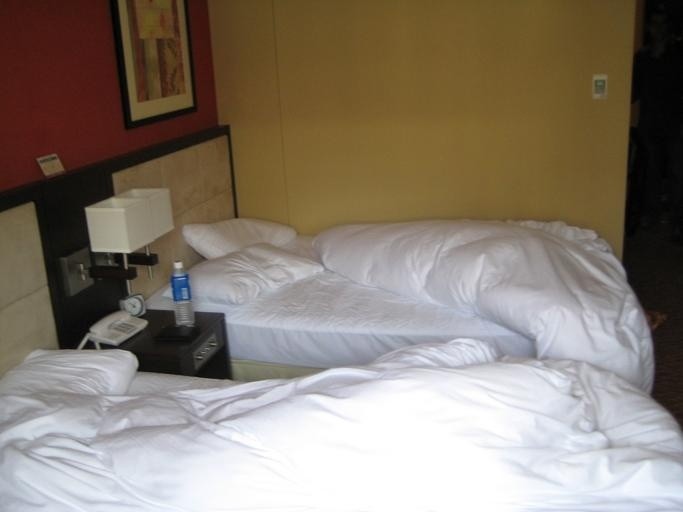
[56,187,175,298]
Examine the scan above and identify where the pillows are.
[160,241,325,305]
[180,218,297,260]
[1,346,140,396]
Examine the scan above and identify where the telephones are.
[88,310,149,346]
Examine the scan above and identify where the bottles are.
[168,260,196,328]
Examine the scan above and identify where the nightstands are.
[72,308,231,378]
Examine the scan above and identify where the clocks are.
[117,294,147,318]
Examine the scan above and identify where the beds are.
[81,124,537,367]
[1,181,682,510]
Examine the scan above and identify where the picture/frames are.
[108,1,200,131]
